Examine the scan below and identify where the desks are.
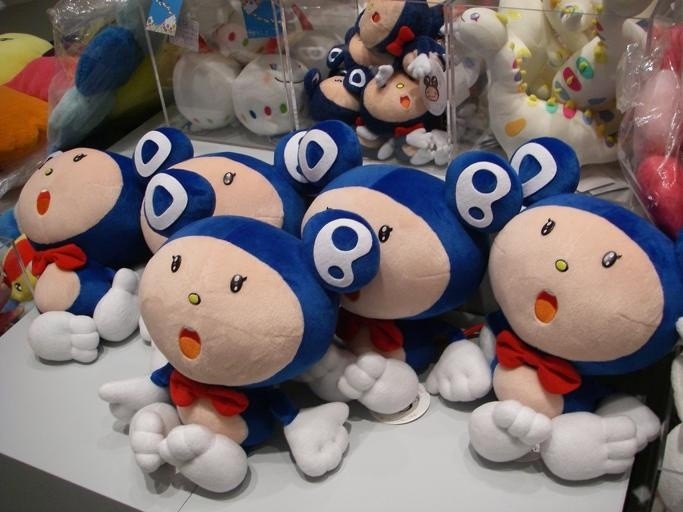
[0,101,646,511]
